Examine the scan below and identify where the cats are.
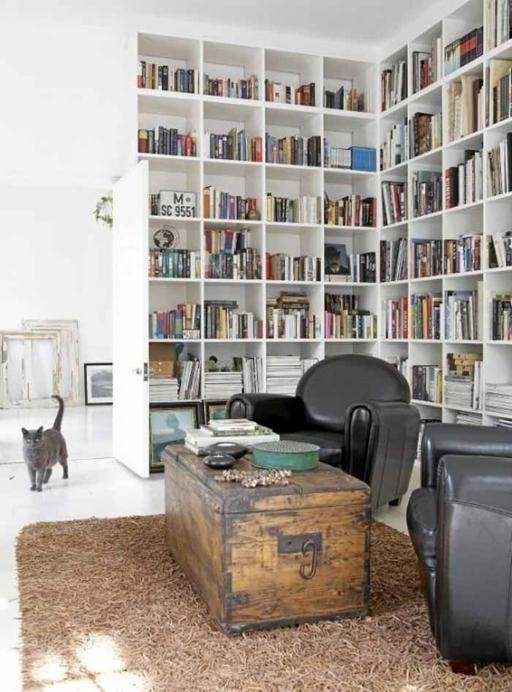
[22,394,70,493]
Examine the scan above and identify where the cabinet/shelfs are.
[376,0,512,457]
[137,17,376,480]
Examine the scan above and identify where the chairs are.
[405,422,511,675]
[226,354,420,508]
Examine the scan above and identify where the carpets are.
[15,514,511,692]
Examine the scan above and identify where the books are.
[202,73,263,400]
[414,37,442,401]
[487,1,512,427]
[136,60,200,402]
[324,85,378,339]
[383,59,406,375]
[185,418,280,456]
[265,79,321,394]
[447,27,483,427]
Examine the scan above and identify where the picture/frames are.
[148,399,202,473]
[203,397,232,427]
[84,363,113,405]
